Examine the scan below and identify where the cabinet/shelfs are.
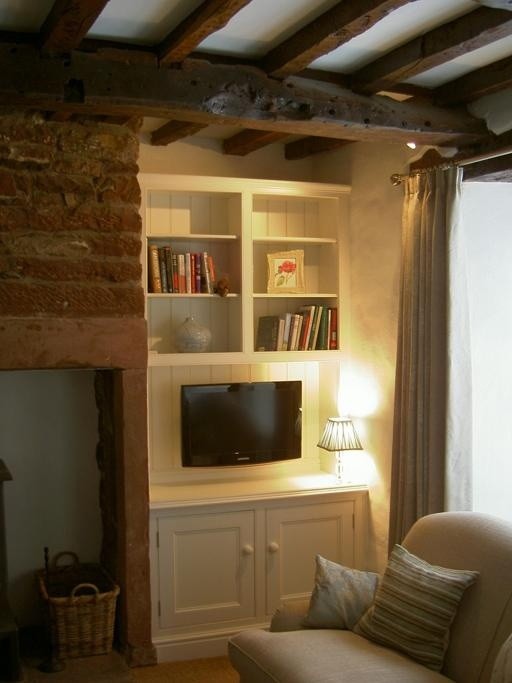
[136,169,370,663]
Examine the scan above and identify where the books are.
[255,305,338,350]
[148,244,217,294]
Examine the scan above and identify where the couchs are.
[228,510,512,683]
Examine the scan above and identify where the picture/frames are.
[265,249,306,294]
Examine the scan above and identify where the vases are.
[176,316,213,354]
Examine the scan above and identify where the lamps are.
[315,416,366,488]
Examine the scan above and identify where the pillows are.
[300,551,384,632]
[356,540,486,675]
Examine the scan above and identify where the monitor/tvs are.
[181,380,302,467]
